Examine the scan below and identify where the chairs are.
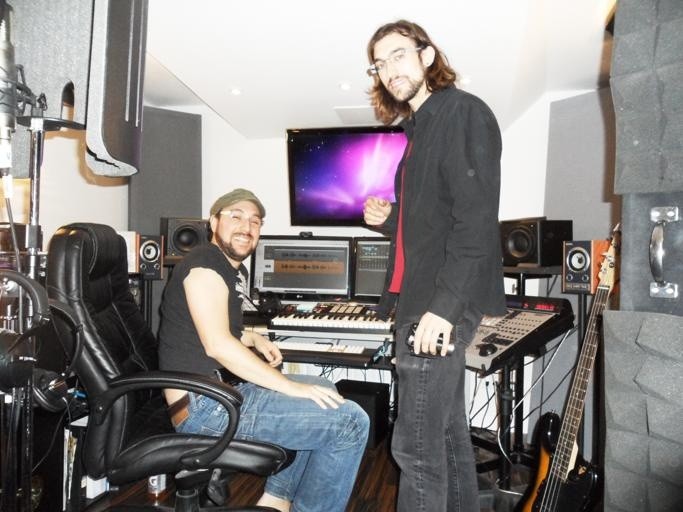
[47,223,297,512]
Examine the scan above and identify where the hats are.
[207,188,267,220]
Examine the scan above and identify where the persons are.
[155,189,371,512]
[362,18,508,511]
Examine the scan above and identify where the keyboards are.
[272,340,365,354]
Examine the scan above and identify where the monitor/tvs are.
[351,237,392,302]
[250,235,353,302]
[287,125,408,227]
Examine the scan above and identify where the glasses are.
[364,45,426,78]
[218,208,262,227]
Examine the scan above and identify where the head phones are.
[0,268,52,388]
[10,298,84,414]
[245,291,282,320]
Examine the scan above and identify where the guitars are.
[512,222,621,511]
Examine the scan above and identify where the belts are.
[165,392,203,418]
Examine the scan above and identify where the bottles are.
[146,473,167,504]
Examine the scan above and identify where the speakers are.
[562,240,620,294]
[335,380,389,448]
[160,216,212,265]
[128,235,165,280]
[499,219,573,274]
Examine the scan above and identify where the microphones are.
[0,3,17,200]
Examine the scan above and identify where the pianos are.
[268,302,394,343]
